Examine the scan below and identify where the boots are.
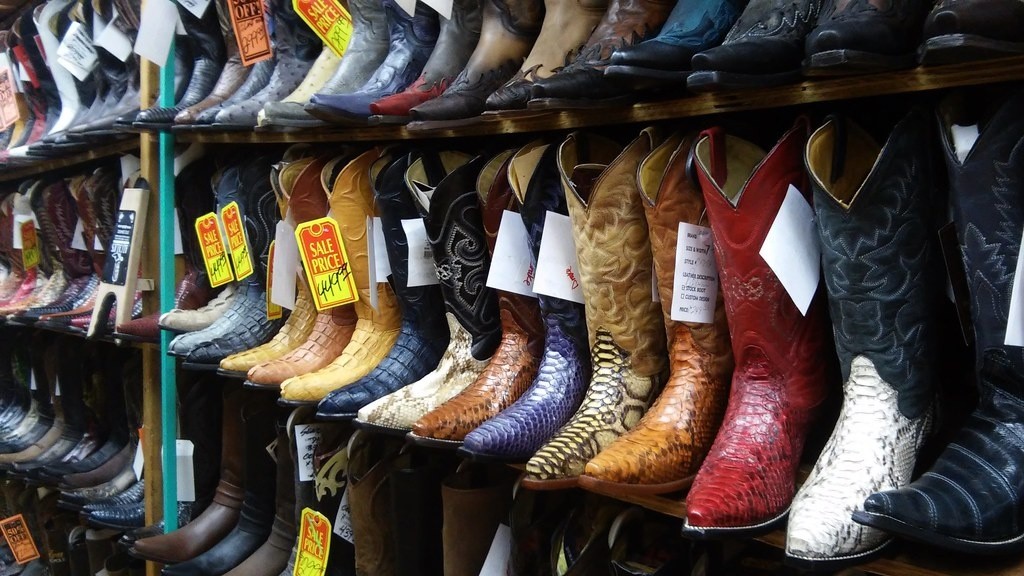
[1,0,1023,575]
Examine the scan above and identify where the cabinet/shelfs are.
[0,0,1024,576]
